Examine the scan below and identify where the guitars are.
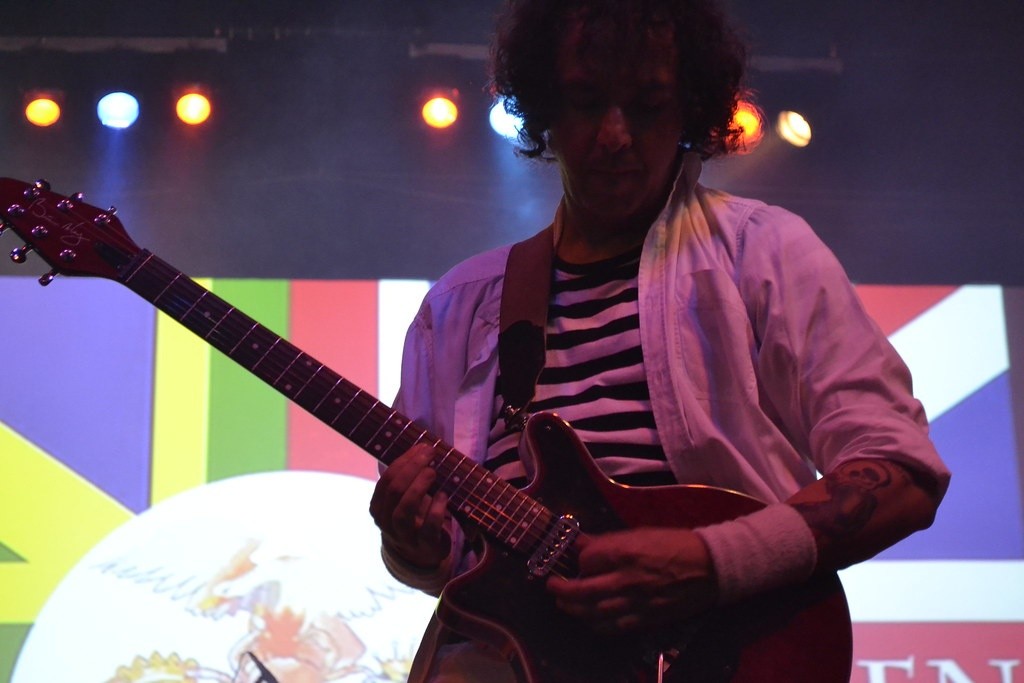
[1,174,853,682]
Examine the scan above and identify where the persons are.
[366,0,951,682]
